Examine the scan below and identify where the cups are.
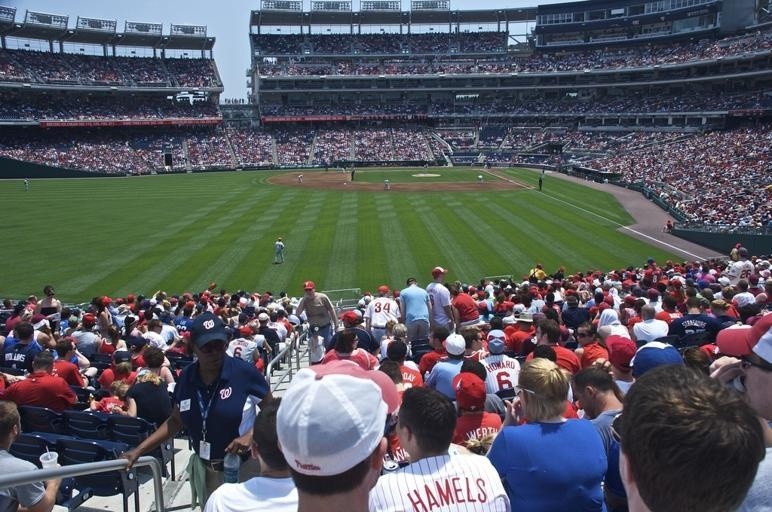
[40,452,59,470]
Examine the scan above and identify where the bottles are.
[224,452,244,484]
[424,371,430,381]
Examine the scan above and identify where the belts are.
[201,457,246,473]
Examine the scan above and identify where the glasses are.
[513,385,534,395]
[610,413,626,444]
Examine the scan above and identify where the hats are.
[303,281,315,290]
[586,263,772,378]
[337,264,590,356]
[736,243,749,256]
[453,373,486,410]
[68,290,293,363]
[276,358,399,476]
[311,324,321,336]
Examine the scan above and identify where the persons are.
[633,25,772,233]
[384,179,391,191]
[537,173,544,194]
[0,241,772,511]
[24,178,29,192]
[2,34,632,186]
[272,237,286,265]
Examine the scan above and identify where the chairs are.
[1,398,158,502]
